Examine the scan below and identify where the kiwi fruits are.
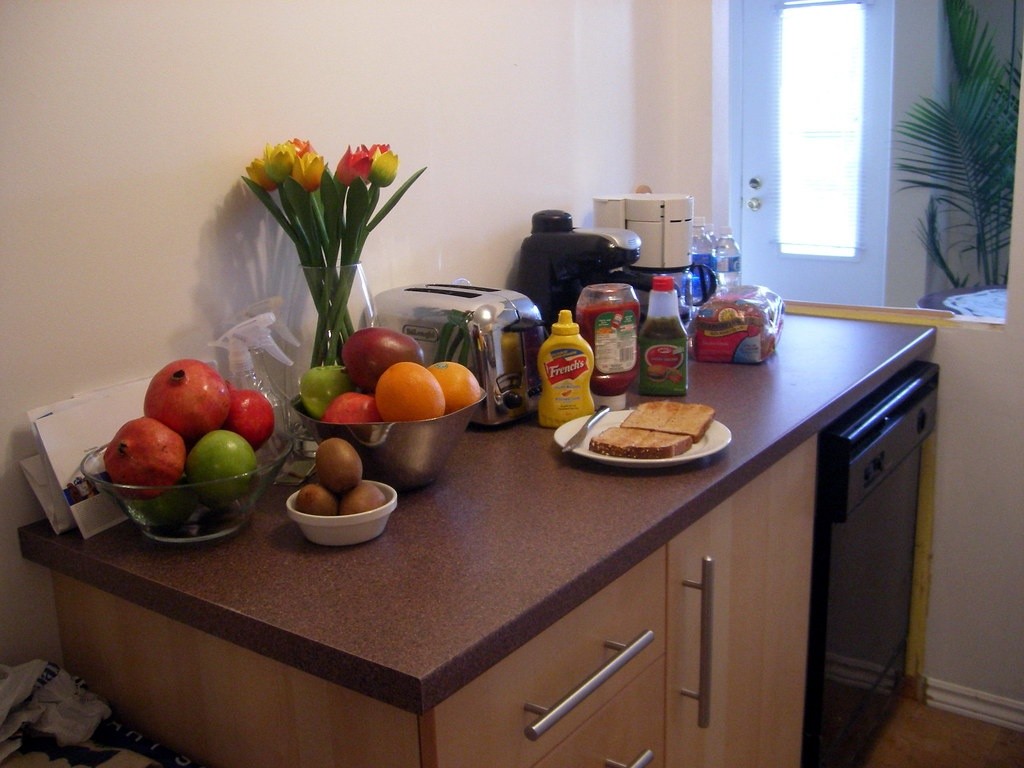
[295,438,387,515]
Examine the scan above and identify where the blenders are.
[591,192,694,323]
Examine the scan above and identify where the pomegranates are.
[104,359,273,494]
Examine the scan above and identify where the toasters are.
[364,282,545,427]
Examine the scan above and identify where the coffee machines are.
[514,209,641,339]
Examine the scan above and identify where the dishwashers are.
[802,360,940,768]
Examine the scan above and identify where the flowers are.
[241,133,426,274]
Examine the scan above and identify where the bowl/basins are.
[80,431,294,541]
[290,387,487,494]
[286,480,397,545]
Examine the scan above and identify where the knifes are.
[561,405,610,454]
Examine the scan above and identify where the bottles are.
[576,283,639,411]
[714,223,740,294]
[538,310,595,428]
[638,276,690,396]
[680,215,716,306]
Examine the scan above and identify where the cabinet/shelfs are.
[666,438,824,768]
[48,549,678,768]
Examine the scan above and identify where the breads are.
[589,400,716,459]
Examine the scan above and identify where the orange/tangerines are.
[375,361,480,421]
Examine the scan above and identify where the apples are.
[123,430,256,527]
[300,364,379,425]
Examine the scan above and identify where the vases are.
[283,263,377,437]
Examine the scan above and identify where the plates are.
[556,409,733,467]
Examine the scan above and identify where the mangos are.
[340,327,422,392]
